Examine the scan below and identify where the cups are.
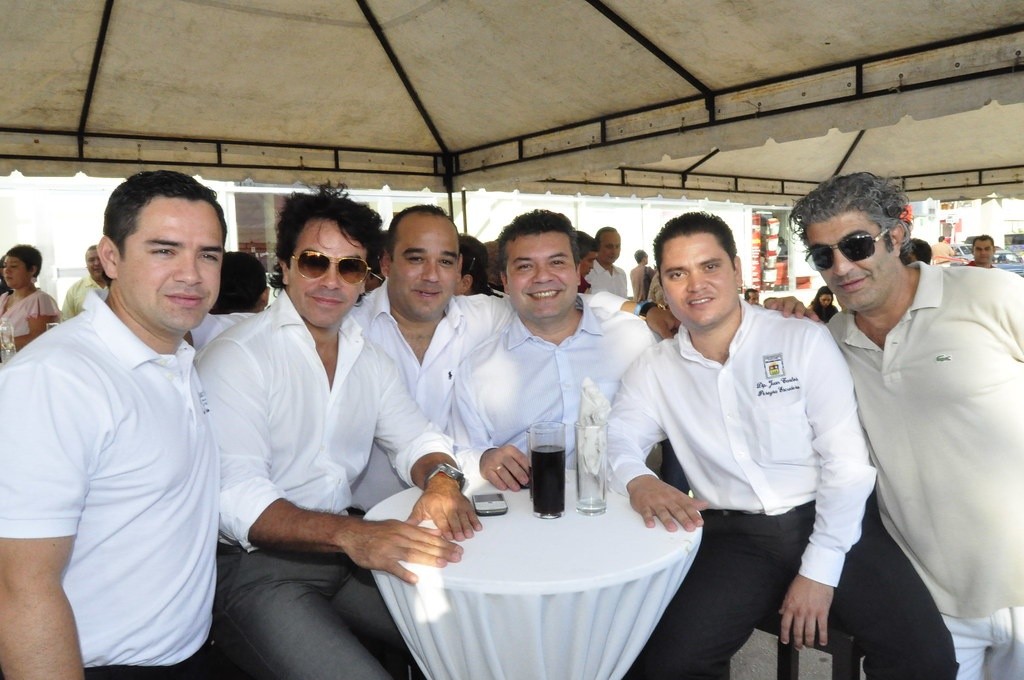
[528,421,568,518]
[45,322,59,331]
[574,423,608,514]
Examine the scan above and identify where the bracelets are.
[634,299,654,315]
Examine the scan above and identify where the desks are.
[361,474,704,680]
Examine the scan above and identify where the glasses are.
[297,245,374,286]
[803,227,895,272]
[821,296,832,302]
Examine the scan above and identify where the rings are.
[493,465,503,471]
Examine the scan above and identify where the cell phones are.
[472,493,508,516]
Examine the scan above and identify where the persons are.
[445,209,671,492]
[186,202,682,521]
[1,170,228,680]
[0,227,996,363]
[195,179,484,680]
[604,212,960,680]
[764,170,1024,680]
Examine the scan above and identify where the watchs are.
[638,302,666,321]
[423,462,465,490]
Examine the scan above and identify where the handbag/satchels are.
[642,266,651,301]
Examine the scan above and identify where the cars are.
[992,250,1024,277]
[949,244,976,261]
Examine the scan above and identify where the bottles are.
[0,316,17,363]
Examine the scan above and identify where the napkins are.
[577,375,612,474]
